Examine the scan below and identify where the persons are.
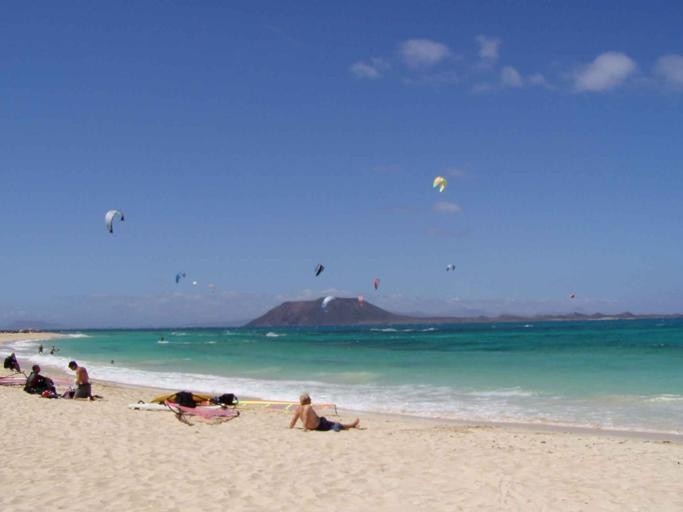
[25,364,58,395]
[4,352,21,373]
[37,344,56,356]
[68,360,98,401]
[286,392,361,432]
